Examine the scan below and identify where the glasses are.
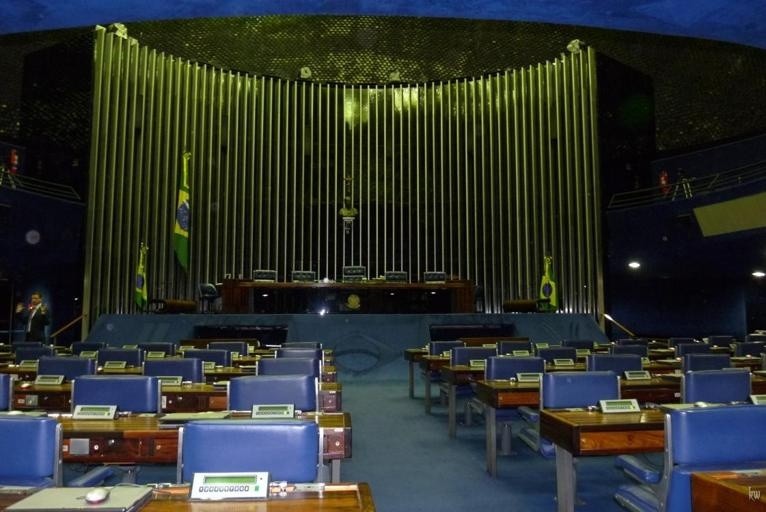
[338,173,359,236]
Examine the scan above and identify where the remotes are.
[85,488,109,503]
[694,401,707,408]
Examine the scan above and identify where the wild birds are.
[4,486,153,512]
[657,404,726,418]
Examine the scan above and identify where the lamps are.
[198,265,485,314]
[338,173,359,236]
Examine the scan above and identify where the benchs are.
[169,151,192,272]
[535,254,560,314]
[133,241,149,310]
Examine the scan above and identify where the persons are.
[15,291,52,343]
[337,195,359,216]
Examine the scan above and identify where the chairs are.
[0,338,379,511]
[198,265,485,314]
[403,334,766,511]
